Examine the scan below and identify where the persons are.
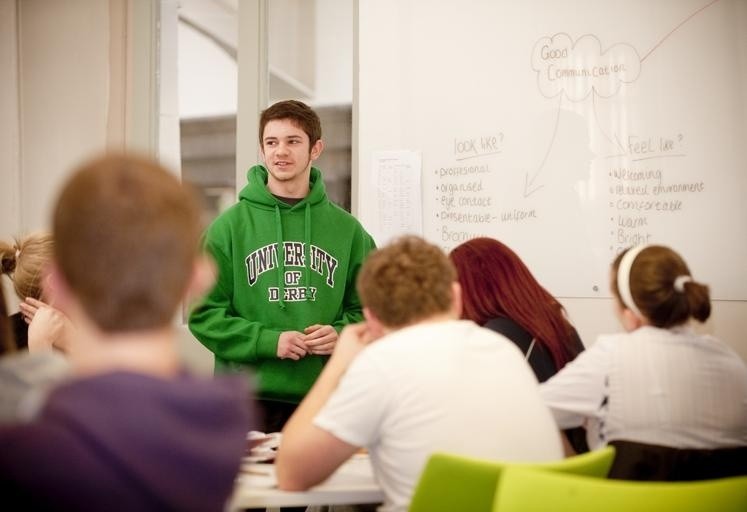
[446,237,610,460]
[185,105,376,431]
[1,231,216,425]
[272,234,577,508]
[2,153,259,510]
[539,243,747,478]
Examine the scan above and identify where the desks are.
[224,452,384,511]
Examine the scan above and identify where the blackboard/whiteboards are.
[351,0,746,303]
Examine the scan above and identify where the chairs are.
[408,443,747,512]
